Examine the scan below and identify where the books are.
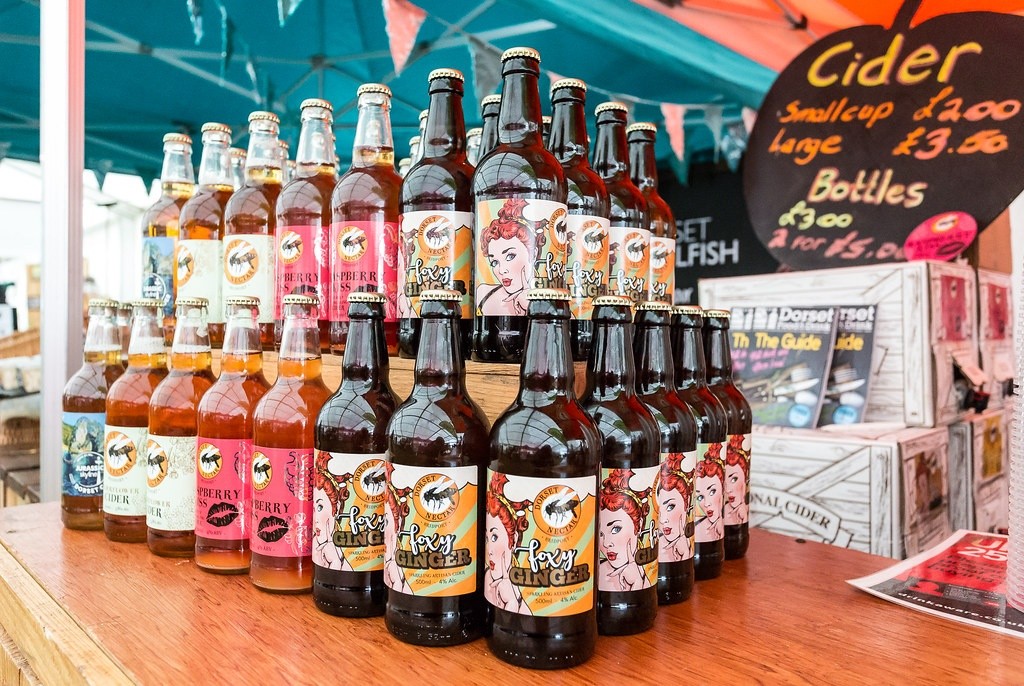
[728,304,878,431]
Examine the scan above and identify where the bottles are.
[550,79,611,361]
[385,289,491,647]
[147,298,218,557]
[399,69,476,358]
[176,121,246,349]
[313,292,404,618]
[486,289,603,670]
[194,295,272,571]
[466,47,568,364]
[577,296,661,637]
[223,111,295,350]
[102,298,170,542]
[143,133,195,347]
[625,122,676,324]
[250,294,333,589]
[61,298,133,531]
[634,302,752,606]
[276,98,341,354]
[329,84,401,356]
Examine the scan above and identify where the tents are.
[0,0,778,502]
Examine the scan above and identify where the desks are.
[1,500,1024,685]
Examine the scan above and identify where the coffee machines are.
[594,102,650,345]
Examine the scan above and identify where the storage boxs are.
[697,259,1013,562]
